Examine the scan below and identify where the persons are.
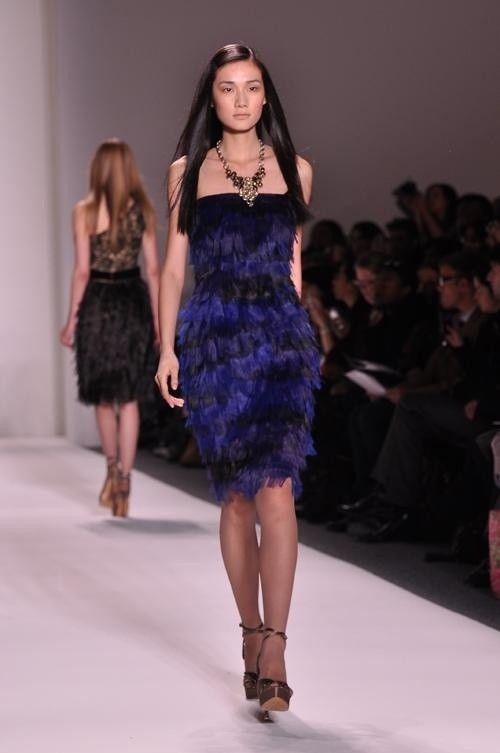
[62,136,162,515]
[153,44,314,723]
[161,177,500,600]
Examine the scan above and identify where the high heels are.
[100,461,129,516]
[240,623,293,720]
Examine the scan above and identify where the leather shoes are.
[330,495,413,540]
[427,527,490,585]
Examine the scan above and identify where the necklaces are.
[214,137,264,210]
